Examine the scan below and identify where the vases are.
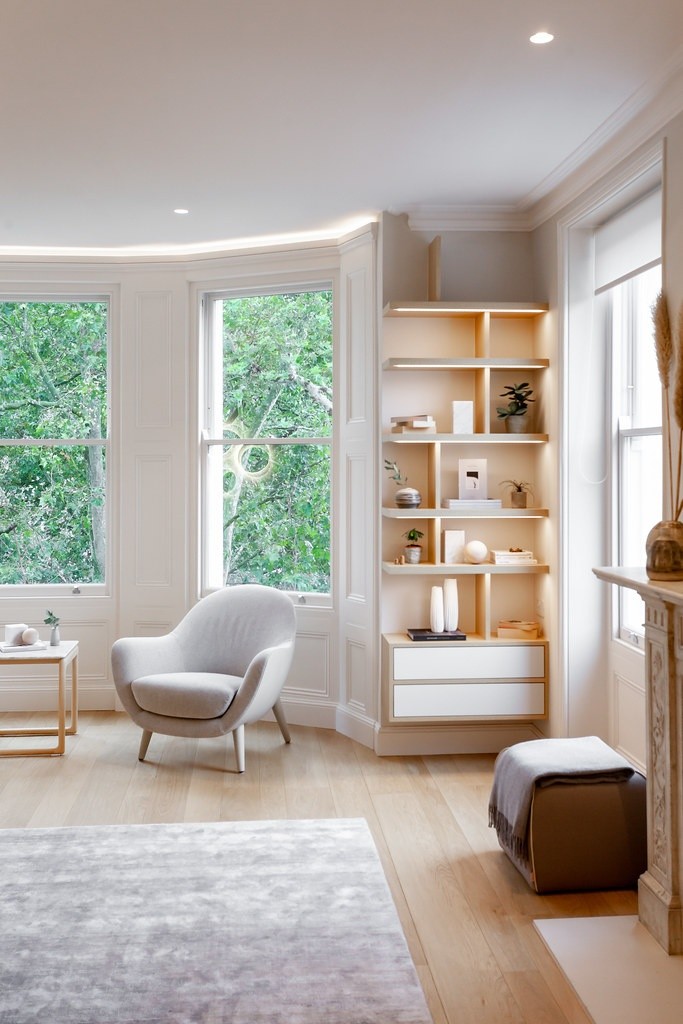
[645,518,683,580]
[51,626,61,646]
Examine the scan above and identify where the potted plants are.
[402,526,425,564]
[385,459,422,508]
[496,381,536,432]
[499,478,536,509]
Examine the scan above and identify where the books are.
[488,549,537,565]
[407,628,468,641]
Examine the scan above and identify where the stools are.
[486,740,656,894]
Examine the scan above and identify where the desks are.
[0,641,80,758]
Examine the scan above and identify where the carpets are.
[0,818,434,1023]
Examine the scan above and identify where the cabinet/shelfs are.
[383,296,554,719]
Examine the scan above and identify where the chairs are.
[108,582,297,772]
